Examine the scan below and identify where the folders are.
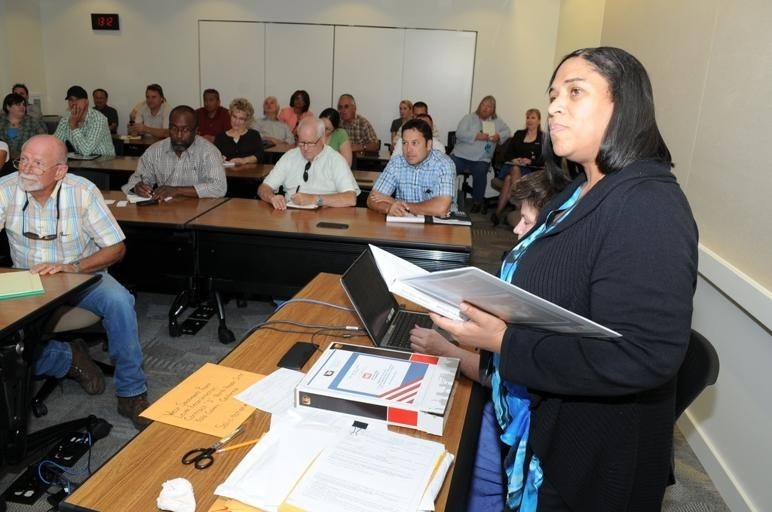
[368,243,622,338]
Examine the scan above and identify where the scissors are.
[181,427,246,470]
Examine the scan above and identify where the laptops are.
[339,248,453,352]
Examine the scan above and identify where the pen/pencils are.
[140,174,144,184]
[405,208,417,217]
[215,439,258,453]
[296,186,300,193]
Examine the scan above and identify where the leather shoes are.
[469,203,500,228]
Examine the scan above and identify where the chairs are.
[491,137,513,191]
[675,328,719,420]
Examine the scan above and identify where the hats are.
[65,85,88,101]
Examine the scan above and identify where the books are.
[431,212,472,225]
[386,214,426,223]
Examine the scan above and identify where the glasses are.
[13,158,59,176]
[298,137,321,147]
[304,160,311,181]
[22,210,59,241]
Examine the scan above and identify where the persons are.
[367,119,458,217]
[493,109,550,217]
[122,102,228,199]
[0,84,446,167]
[449,95,510,214]
[0,134,155,431]
[409,168,574,512]
[257,116,363,211]
[427,47,698,512]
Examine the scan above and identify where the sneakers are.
[68,339,105,394]
[118,392,152,431]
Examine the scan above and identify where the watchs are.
[71,261,80,272]
[318,194,323,207]
